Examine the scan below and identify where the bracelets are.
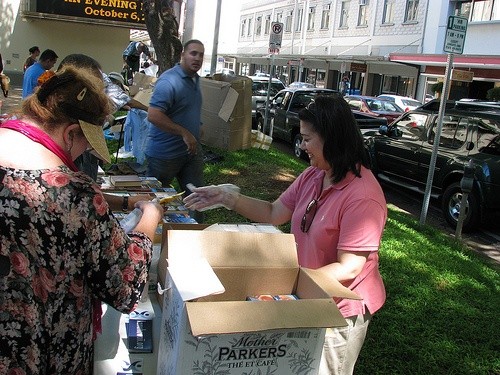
[122,197,129,214]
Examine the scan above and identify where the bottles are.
[119,208,143,233]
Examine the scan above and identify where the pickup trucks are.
[255,87,389,161]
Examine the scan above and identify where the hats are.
[108,72,126,91]
[54,100,112,165]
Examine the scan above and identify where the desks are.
[94,175,199,375]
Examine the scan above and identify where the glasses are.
[299,199,317,233]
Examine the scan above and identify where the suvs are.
[360,98,500,232]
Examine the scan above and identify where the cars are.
[286,82,315,88]
[455,98,482,111]
[252,73,270,77]
[424,93,434,103]
[344,95,405,125]
[219,68,235,75]
[378,94,423,113]
[247,76,286,124]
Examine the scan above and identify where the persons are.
[143,40,205,223]
[183,96,386,375]
[56,53,154,185]
[0,64,164,375]
[23,46,55,102]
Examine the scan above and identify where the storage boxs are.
[199,73,253,153]
[251,130,273,151]
[129,72,158,111]
[157,223,363,375]
